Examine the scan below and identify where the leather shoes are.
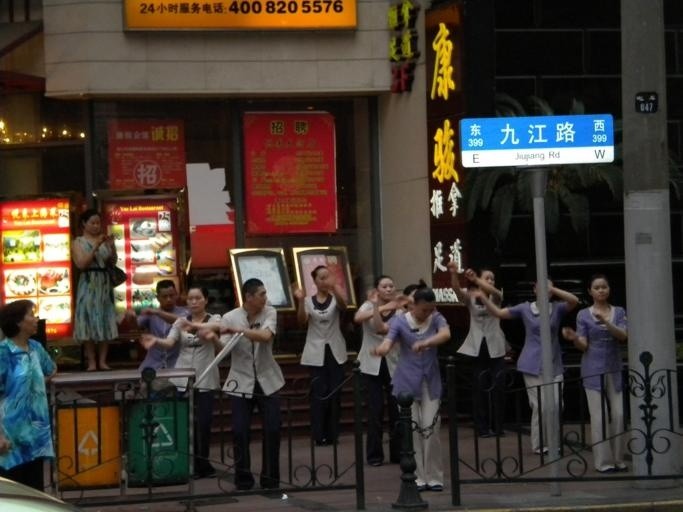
[86,363,114,372]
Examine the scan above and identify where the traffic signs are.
[459,112,614,167]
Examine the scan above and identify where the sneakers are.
[478,427,504,438]
[598,467,628,473]
[370,460,381,466]
[417,485,442,491]
[536,448,561,454]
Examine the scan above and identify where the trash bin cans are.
[50,368,196,491]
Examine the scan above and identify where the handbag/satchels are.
[109,265,125,287]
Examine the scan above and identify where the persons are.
[138,284,223,479]
[465,274,580,456]
[447,259,509,438]
[288,265,350,446]
[353,273,415,466]
[69,208,118,373]
[561,272,630,473]
[0,299,58,492]
[124,279,189,369]
[196,277,284,500]
[398,282,423,312]
[368,286,451,493]
